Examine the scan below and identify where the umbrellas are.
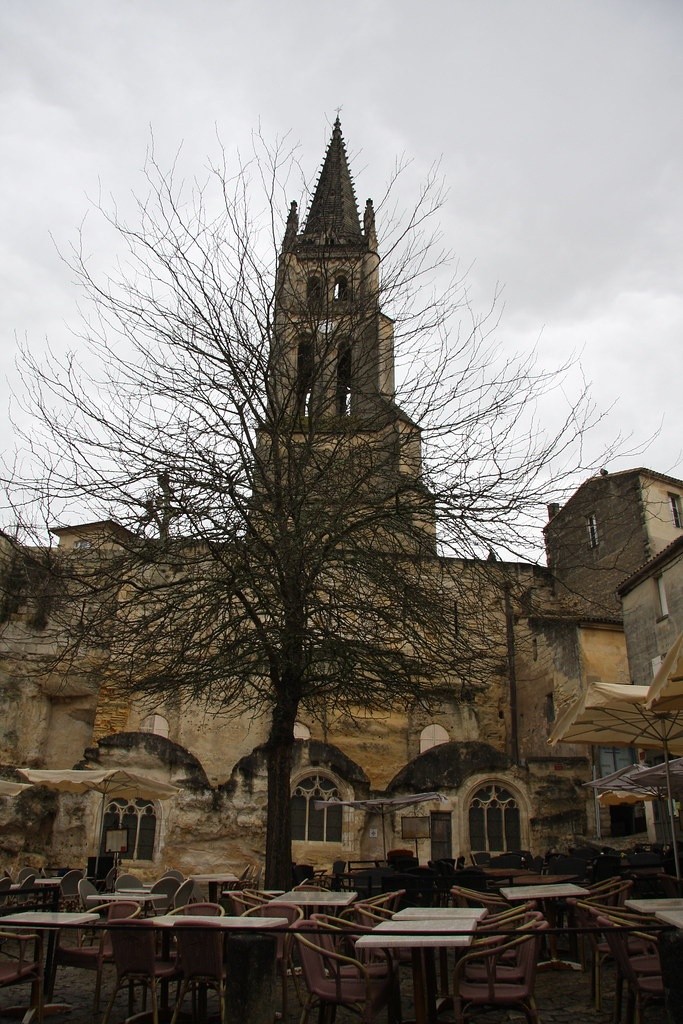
[546,628,683,881]
[15,769,185,880]
[314,792,450,868]
[1,780,34,797]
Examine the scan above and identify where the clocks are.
[317,318,333,333]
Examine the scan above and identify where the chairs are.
[0,840,683,1024]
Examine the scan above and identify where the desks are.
[86,892,167,900]
[482,867,538,885]
[21,878,62,885]
[654,909,682,929]
[623,896,683,913]
[141,912,287,1024]
[269,889,357,921]
[495,873,579,882]
[497,882,589,970]
[0,910,100,1024]
[189,873,238,902]
[117,888,150,893]
[392,906,487,1007]
[356,920,478,1023]
[8,883,19,890]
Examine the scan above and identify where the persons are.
[333,854,347,877]
